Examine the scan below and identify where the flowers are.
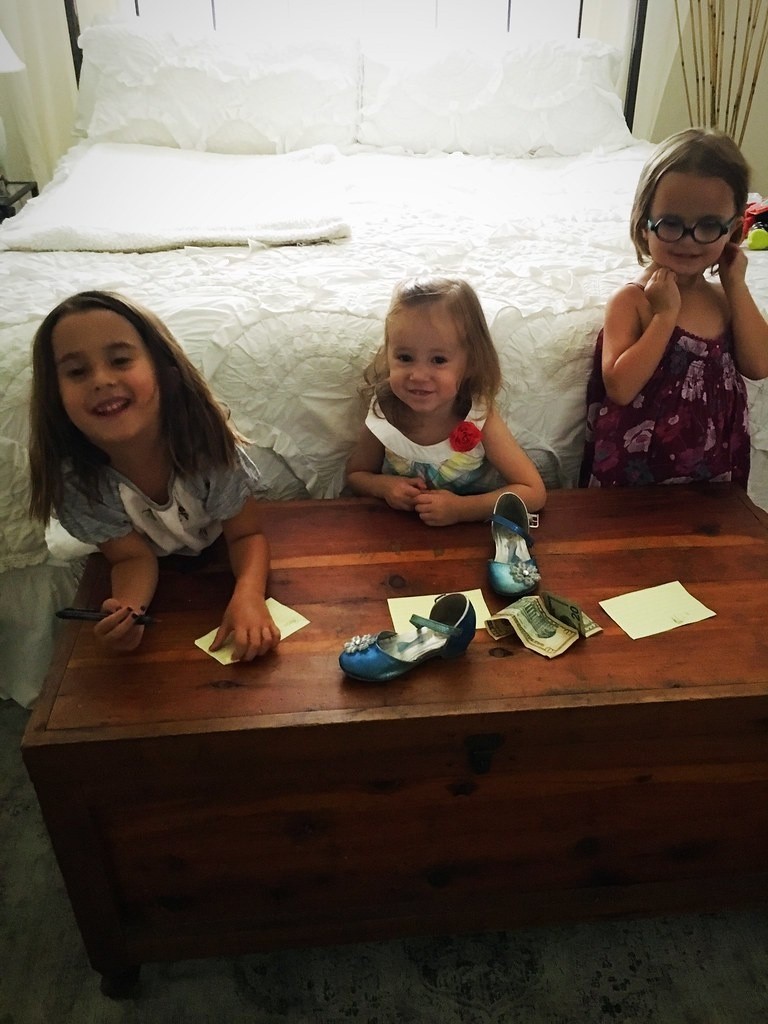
[450,421,484,452]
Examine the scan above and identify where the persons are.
[349,272,545,528]
[577,129,767,489]
[30,292,280,661]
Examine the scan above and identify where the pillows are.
[354,28,640,158]
[85,11,361,156]
[68,24,114,137]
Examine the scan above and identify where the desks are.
[20,479,767,996]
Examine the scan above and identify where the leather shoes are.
[340,593,476,683]
[489,491,542,596]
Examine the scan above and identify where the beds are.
[1,139,766,720]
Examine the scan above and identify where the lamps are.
[1,28,26,198]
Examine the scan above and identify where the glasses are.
[648,213,738,245]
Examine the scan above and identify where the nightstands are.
[0,181,40,223]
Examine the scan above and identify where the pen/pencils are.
[55,607,163,625]
